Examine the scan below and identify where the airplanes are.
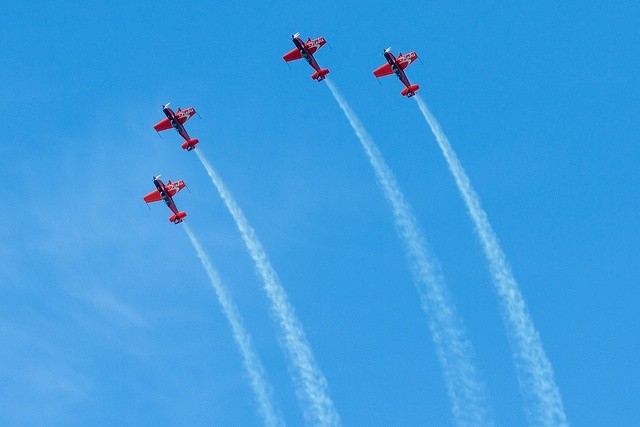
[283,32,330,82]
[142,174,188,225]
[153,103,199,153]
[374,47,420,98]
